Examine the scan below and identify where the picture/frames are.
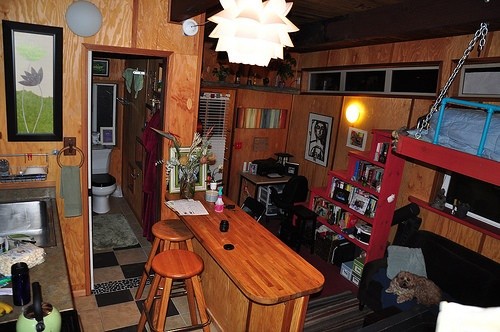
[3,19,64,143]
[92,58,110,77]
[169,144,207,194]
[303,111,335,168]
[345,126,368,151]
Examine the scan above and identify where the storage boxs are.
[339,261,354,281]
[353,258,363,276]
[350,270,360,289]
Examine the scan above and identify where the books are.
[164,200,210,216]
[311,142,390,246]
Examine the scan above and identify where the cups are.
[205,190,218,202]
[456,202,470,217]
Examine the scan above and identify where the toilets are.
[91,143,117,215]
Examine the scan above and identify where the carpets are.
[299,289,367,332]
[92,212,139,249]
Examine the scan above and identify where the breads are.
[0,242,45,276]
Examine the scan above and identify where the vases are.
[180,169,195,199]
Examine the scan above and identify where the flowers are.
[150,125,216,185]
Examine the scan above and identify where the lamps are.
[206,0,300,67]
[345,104,362,122]
[65,1,103,38]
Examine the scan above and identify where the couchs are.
[355,231,500,318]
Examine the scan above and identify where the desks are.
[163,193,326,332]
[236,172,291,217]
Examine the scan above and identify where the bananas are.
[0,301,13,316]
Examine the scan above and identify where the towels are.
[141,110,162,241]
[122,67,144,99]
[59,165,83,218]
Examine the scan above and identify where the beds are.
[394,92,500,187]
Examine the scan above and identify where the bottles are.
[220,220,229,232]
[11,262,31,306]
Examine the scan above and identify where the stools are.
[136,218,207,303]
[137,248,211,332]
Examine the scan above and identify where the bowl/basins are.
[24,167,47,177]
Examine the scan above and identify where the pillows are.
[435,300,500,332]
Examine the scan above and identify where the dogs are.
[385,271,442,304]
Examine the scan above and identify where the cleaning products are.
[214,186,224,213]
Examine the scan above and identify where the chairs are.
[240,196,267,223]
[269,174,315,254]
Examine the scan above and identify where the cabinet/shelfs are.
[307,128,406,263]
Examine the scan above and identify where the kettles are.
[16,281,62,332]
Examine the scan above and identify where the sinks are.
[0,196,63,255]
